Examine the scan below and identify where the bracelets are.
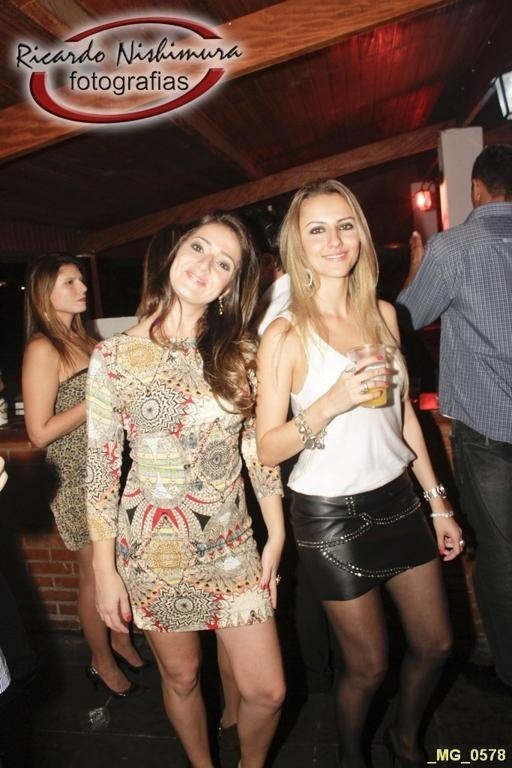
[294,411,325,448]
[423,483,447,501]
[430,510,455,518]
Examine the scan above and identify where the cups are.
[345,342,390,409]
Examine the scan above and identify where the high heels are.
[383,727,429,768]
[85,664,140,699]
[109,644,153,675]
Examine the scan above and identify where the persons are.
[254,178,465,768]
[217,636,240,751]
[248,220,333,695]
[0,381,42,698]
[394,145,511,690]
[21,252,155,699]
[83,214,287,767]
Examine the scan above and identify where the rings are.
[344,361,358,375]
[363,381,369,392]
[274,575,282,585]
[459,540,465,547]
